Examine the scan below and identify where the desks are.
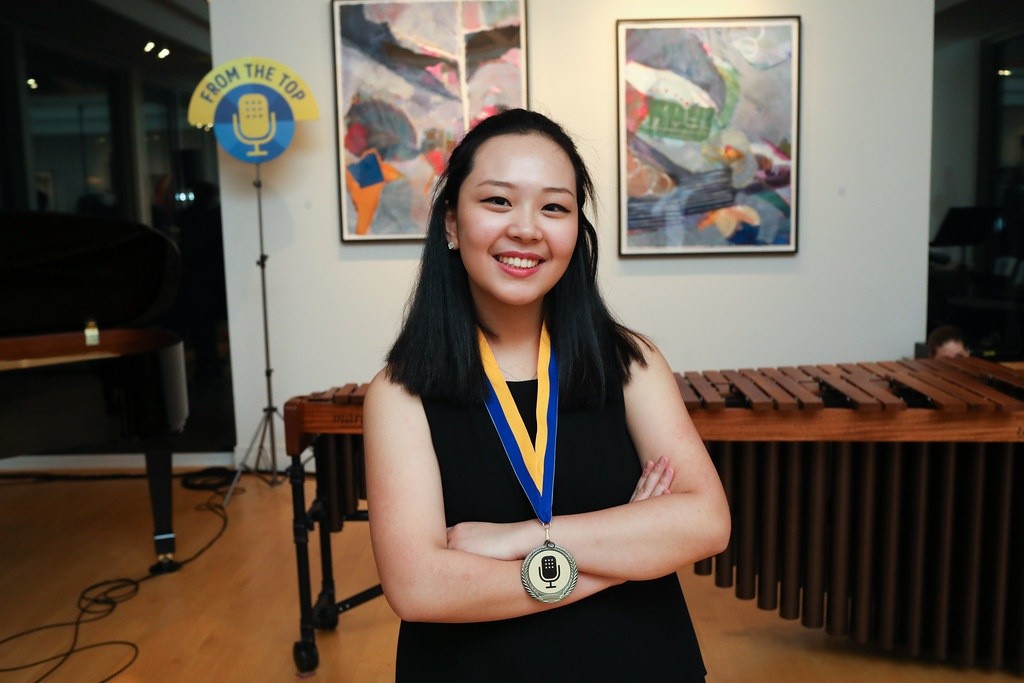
[1,330,184,575]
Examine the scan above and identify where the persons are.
[364,108,730,682]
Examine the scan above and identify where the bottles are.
[85,322,99,346]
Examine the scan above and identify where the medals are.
[522,542,578,603]
